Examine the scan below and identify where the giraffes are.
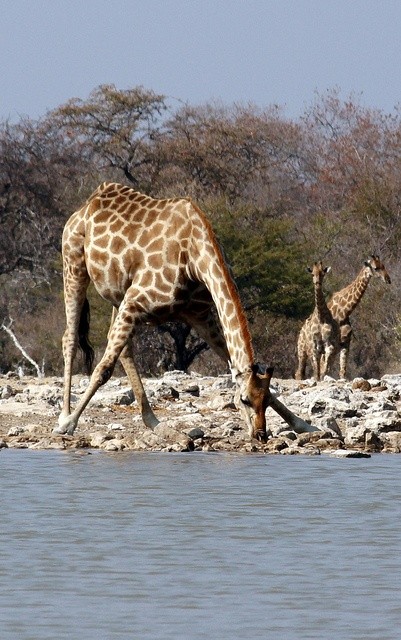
[295,258,342,384]
[53,181,321,442]
[320,254,393,380]
